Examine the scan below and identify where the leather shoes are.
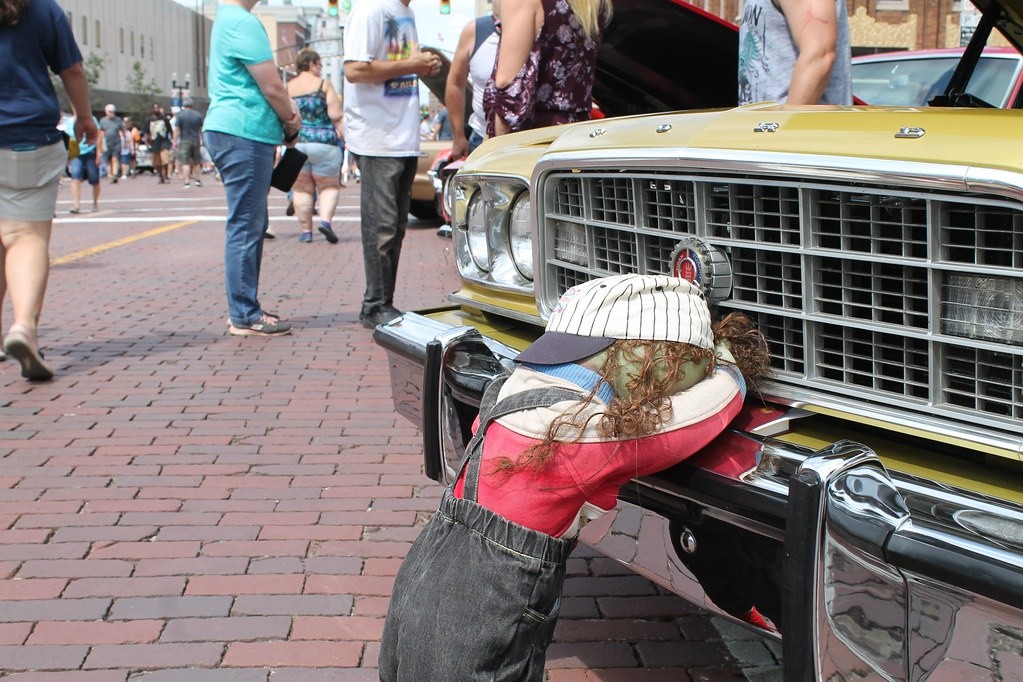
[359,305,402,329]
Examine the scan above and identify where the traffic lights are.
[328,0,338,17]
[440,0,450,15]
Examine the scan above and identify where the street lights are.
[171,72,191,112]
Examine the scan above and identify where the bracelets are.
[98,153,103,156]
[285,131,299,143]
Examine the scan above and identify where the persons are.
[739,0,852,106]
[54,98,222,218]
[265,49,362,244]
[378,273,769,682]
[0,0,98,381]
[202,0,303,337]
[341,0,442,329]
[417,0,613,162]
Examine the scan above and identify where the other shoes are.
[120,175,128,179]
[264,233,276,239]
[356,178,360,184]
[286,201,295,216]
[0,322,54,380]
[182,178,203,189]
[92,203,98,213]
[110,179,118,183]
[70,208,80,213]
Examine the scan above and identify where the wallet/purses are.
[270,147,308,193]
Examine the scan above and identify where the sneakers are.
[318,221,338,244]
[226,310,293,336]
[299,231,313,242]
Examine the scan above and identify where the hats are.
[513,274,715,366]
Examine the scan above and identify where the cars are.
[374,0,1023,682]
